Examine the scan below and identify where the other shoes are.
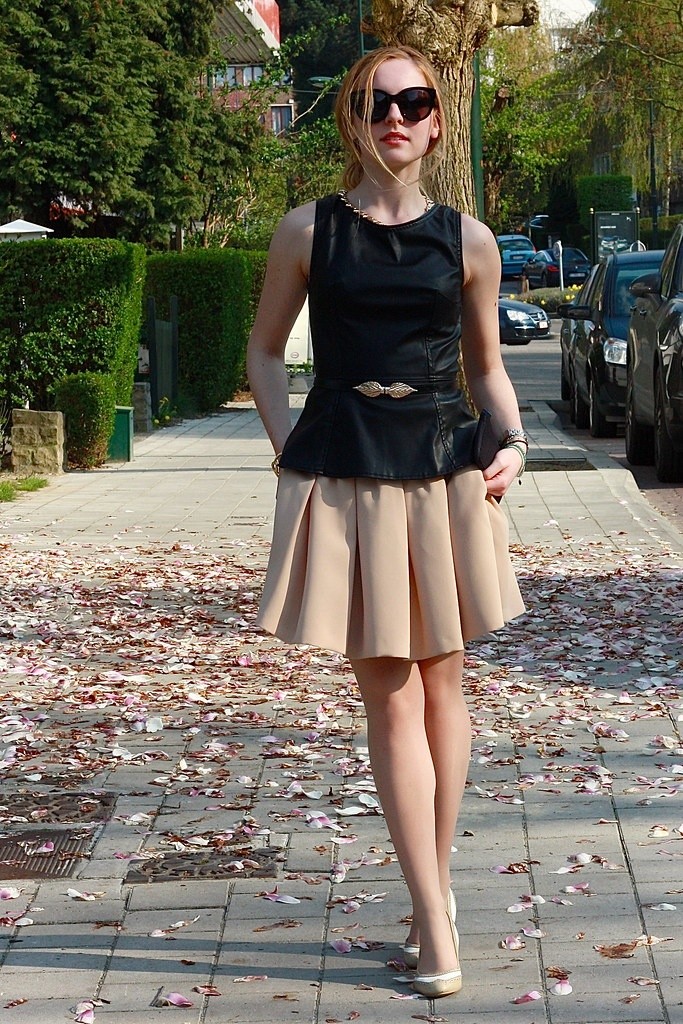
[402,886,463,998]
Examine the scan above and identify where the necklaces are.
[338,187,436,225]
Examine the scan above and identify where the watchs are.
[502,427,529,445]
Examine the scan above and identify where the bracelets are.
[505,439,529,478]
[271,452,282,478]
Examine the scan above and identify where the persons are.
[247,45,529,997]
[522,274,529,293]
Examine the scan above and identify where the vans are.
[497,234,537,282]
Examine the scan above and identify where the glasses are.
[350,86,436,125]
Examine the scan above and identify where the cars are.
[504,216,579,251]
[520,245,593,290]
[556,249,666,439]
[498,298,552,346]
[624,217,683,484]
